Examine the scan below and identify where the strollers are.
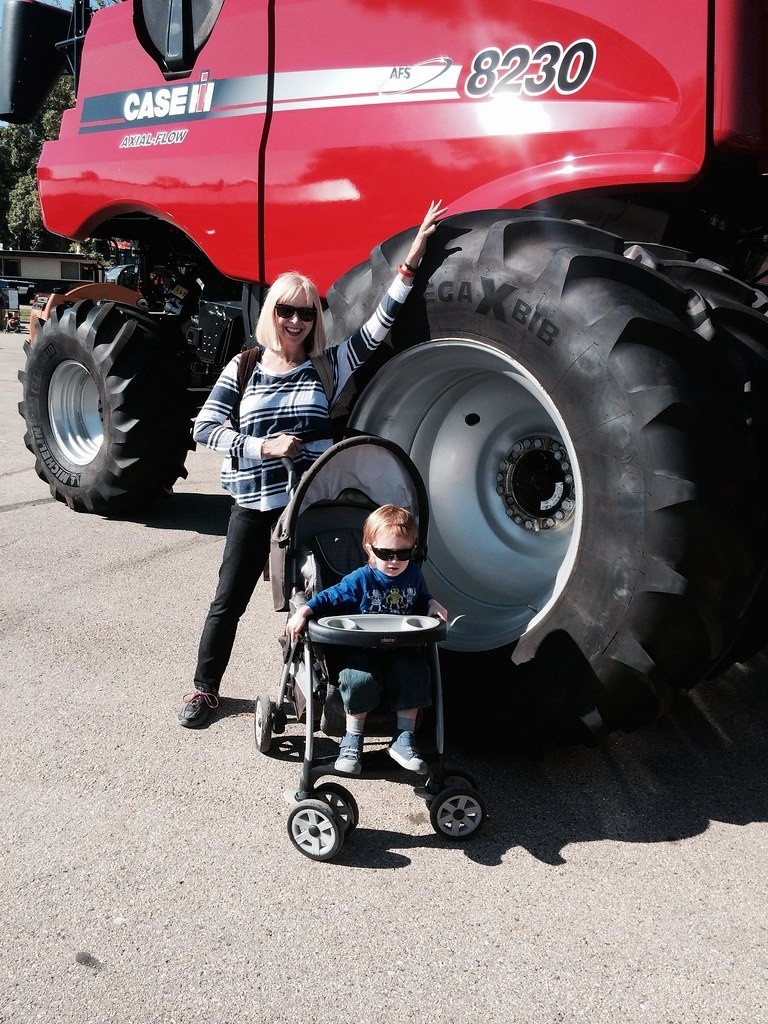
[251,426,490,862]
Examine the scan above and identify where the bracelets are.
[398,262,417,277]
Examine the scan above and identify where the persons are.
[177,199,449,728]
[286,505,448,775]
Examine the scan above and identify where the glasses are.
[274,304,317,322]
[370,543,415,561]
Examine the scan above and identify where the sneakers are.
[387,729,427,772]
[178,689,220,727]
[335,732,364,774]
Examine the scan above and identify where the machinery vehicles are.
[15,1,768,791]
